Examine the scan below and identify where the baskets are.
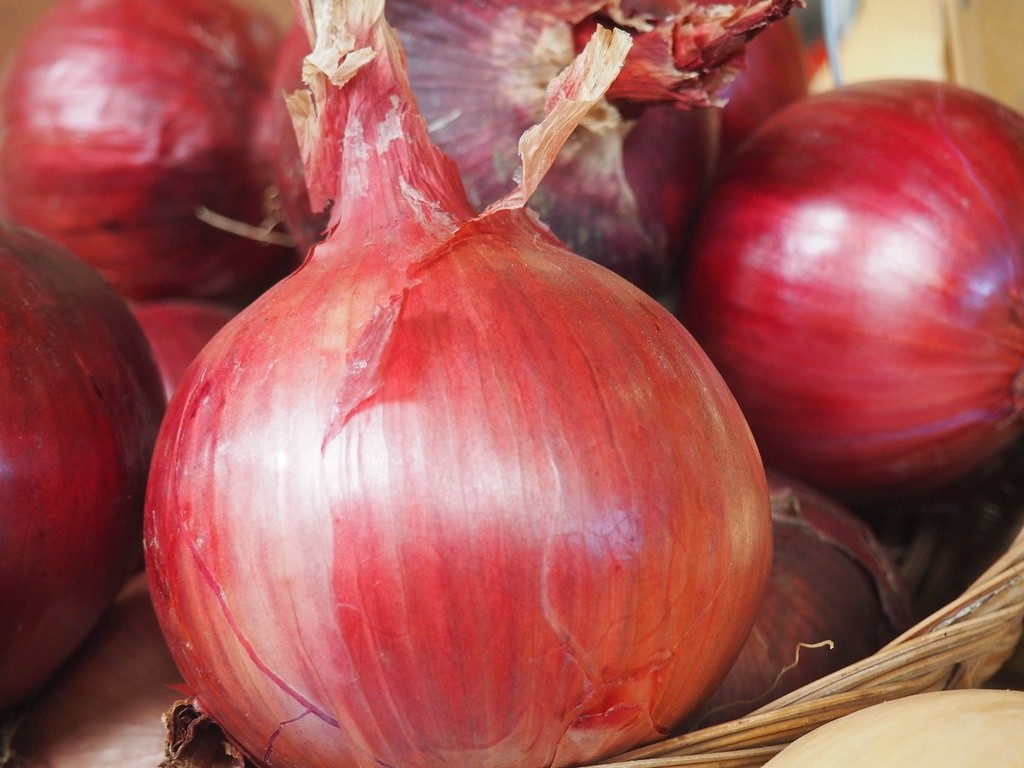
[577,437,1024,768]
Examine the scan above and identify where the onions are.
[0,0,1024,768]
